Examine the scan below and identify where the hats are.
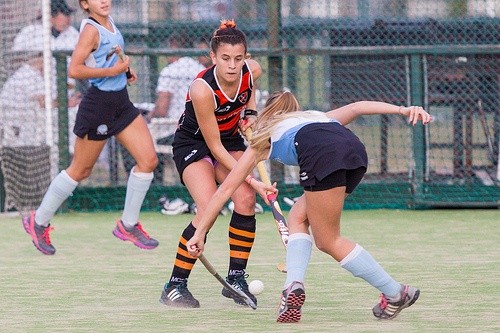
[50,0,73,14]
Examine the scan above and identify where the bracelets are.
[244,109,257,117]
[247,177,254,185]
[398,106,405,116]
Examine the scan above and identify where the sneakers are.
[22,210,55,255]
[112,219,159,249]
[276,281,305,323]
[159,278,200,308]
[372,283,420,319]
[221,270,257,306]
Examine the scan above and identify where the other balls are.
[248,280,264,295]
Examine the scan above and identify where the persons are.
[0,0,83,147]
[121,34,207,185]
[159,19,279,309]
[198,38,213,67]
[22,0,159,255]
[186,88,432,324]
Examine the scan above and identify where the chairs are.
[148,118,183,156]
[3,103,46,147]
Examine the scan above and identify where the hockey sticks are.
[106,45,132,86]
[240,116,290,273]
[191,245,257,310]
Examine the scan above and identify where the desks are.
[107,102,154,181]
